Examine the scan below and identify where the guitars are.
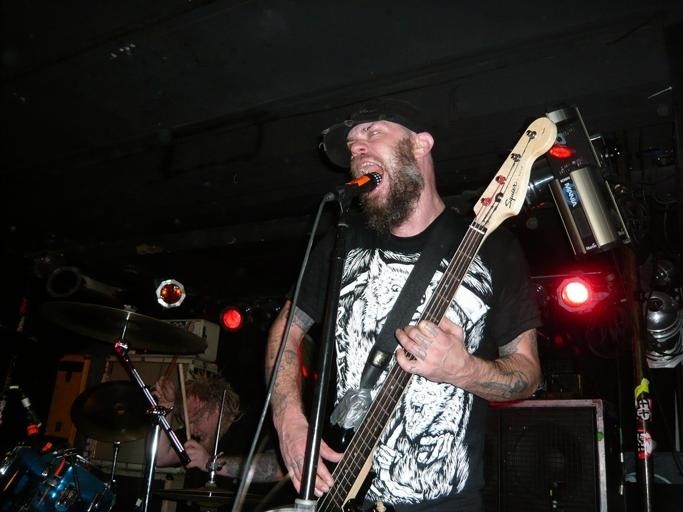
[315,117,557,510]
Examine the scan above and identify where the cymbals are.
[24,299,206,354]
[154,486,260,510]
[70,380,153,441]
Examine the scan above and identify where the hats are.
[323,99,434,167]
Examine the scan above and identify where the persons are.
[265,99,542,512]
[146,371,287,512]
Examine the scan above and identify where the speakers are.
[487,398,626,512]
[624,450,683,512]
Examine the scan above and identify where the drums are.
[0,442,119,510]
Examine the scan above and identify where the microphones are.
[326,172,383,201]
[524,104,633,259]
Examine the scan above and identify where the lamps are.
[638,290,683,372]
